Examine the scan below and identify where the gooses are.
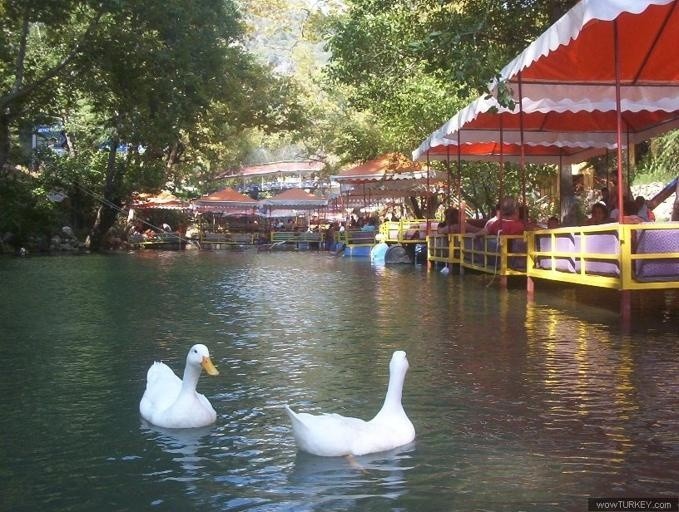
[139,342,220,430]
[281,348,417,474]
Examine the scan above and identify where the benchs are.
[203,220,679,279]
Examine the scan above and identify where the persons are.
[437,195,656,239]
[596,187,609,207]
[131,220,173,242]
[607,169,635,210]
[272,207,416,233]
